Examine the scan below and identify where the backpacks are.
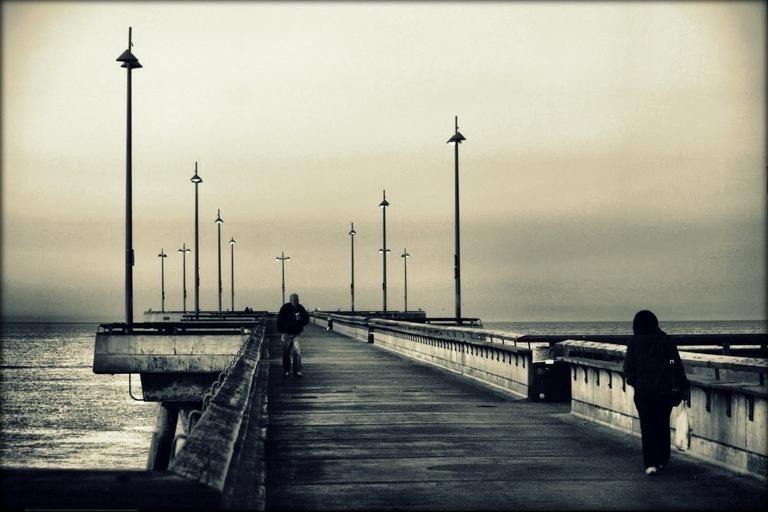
[661,334,687,400]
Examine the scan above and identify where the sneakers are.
[283,370,304,377]
[644,463,670,477]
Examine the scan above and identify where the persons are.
[276,293,310,376]
[621,310,691,472]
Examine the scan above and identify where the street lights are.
[115,25,141,329]
[446,116,466,328]
[158,160,238,313]
[275,251,292,306]
[347,190,410,313]
